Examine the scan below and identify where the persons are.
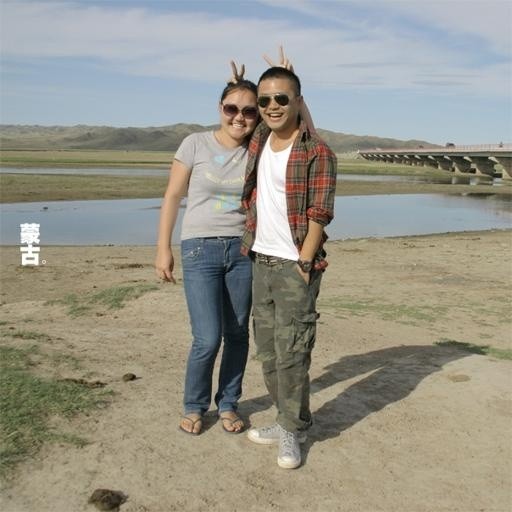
[227,55,338,471]
[151,42,320,435]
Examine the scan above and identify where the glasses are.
[221,102,258,120]
[258,94,290,108]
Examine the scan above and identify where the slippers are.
[220,409,245,433]
[177,413,204,436]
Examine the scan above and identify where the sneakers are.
[247,421,307,445]
[276,424,302,470]
